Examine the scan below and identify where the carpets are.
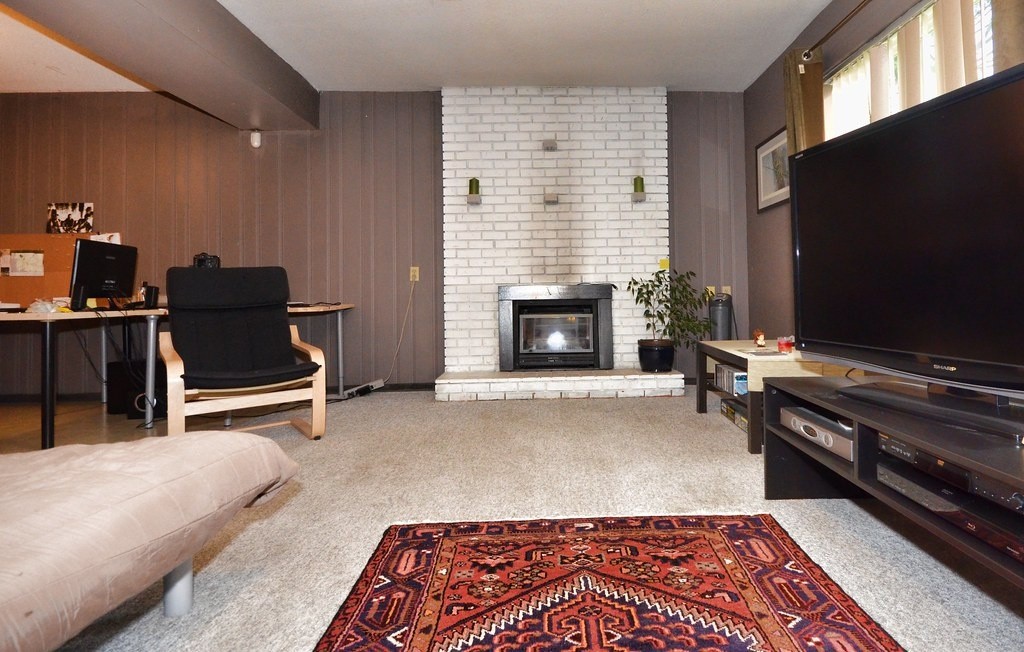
[313,513,908,652]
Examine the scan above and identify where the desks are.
[0,307,170,451]
[285,305,355,399]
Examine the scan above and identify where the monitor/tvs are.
[69,238,138,312]
[787,61,1024,440]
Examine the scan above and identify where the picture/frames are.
[755,125,791,214]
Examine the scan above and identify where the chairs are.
[158,266,327,441]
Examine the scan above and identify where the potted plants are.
[627,271,718,373]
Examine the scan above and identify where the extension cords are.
[344,379,384,398]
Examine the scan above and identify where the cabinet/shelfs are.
[695,340,823,454]
[763,374,1024,591]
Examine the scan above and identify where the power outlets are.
[410,266,419,282]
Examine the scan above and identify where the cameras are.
[194,253,219,269]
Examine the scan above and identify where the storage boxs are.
[0,231,125,309]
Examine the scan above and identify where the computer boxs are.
[106,359,167,414]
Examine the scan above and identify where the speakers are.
[71,281,87,312]
[145,286,159,309]
[127,389,168,419]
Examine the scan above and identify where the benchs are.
[1,430,299,652]
